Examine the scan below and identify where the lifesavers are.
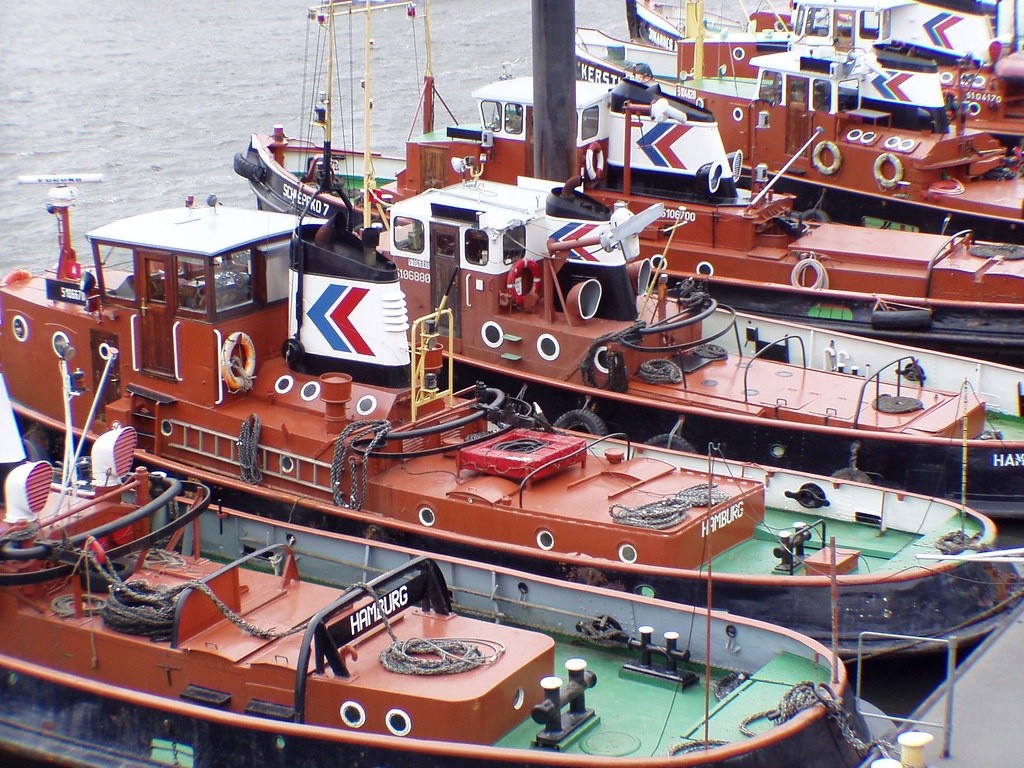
[872,151,903,189]
[813,140,843,176]
[220,330,256,393]
[507,258,542,309]
[78,554,134,591]
[798,209,831,223]
[585,141,604,181]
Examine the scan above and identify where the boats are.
[0,0,1024,768]
